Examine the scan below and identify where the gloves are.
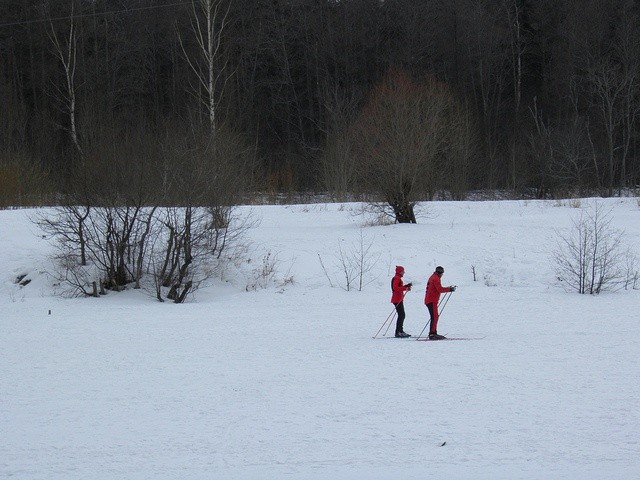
[450,286,456,291]
[408,282,413,286]
[408,287,411,291]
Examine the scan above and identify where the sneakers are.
[428,332,446,340]
[395,332,411,338]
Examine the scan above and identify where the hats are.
[395,265,404,274]
[436,266,444,274]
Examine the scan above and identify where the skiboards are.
[417,336,486,341]
[377,337,428,339]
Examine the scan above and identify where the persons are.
[391,266,411,338]
[423,266,456,339]
